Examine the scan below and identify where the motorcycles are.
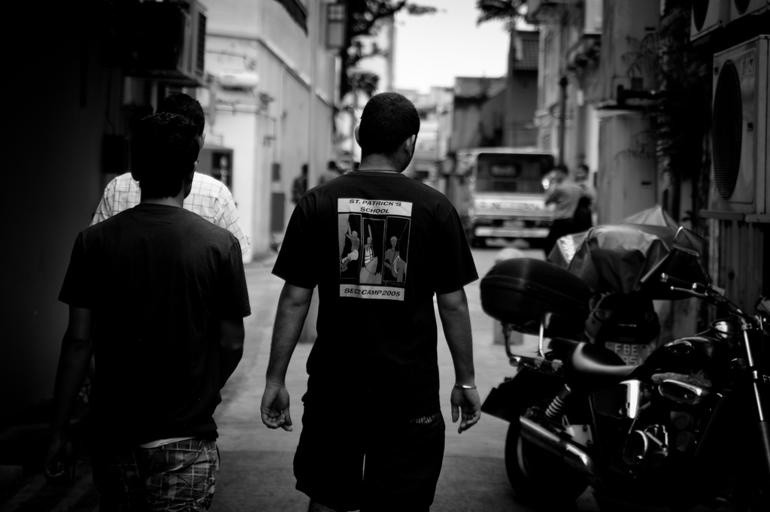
[480,226,770,511]
[551,204,709,369]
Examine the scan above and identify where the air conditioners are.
[689,1,731,44]
[694,33,769,226]
[144,0,212,88]
[728,1,770,26]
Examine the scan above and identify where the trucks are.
[444,146,561,250]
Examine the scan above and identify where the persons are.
[340,221,360,272]
[385,236,406,282]
[261,93,481,512]
[545,164,581,258]
[573,164,594,232]
[290,149,472,243]
[44,112,251,512]
[364,224,378,274]
[89,91,250,256]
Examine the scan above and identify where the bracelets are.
[454,384,476,390]
